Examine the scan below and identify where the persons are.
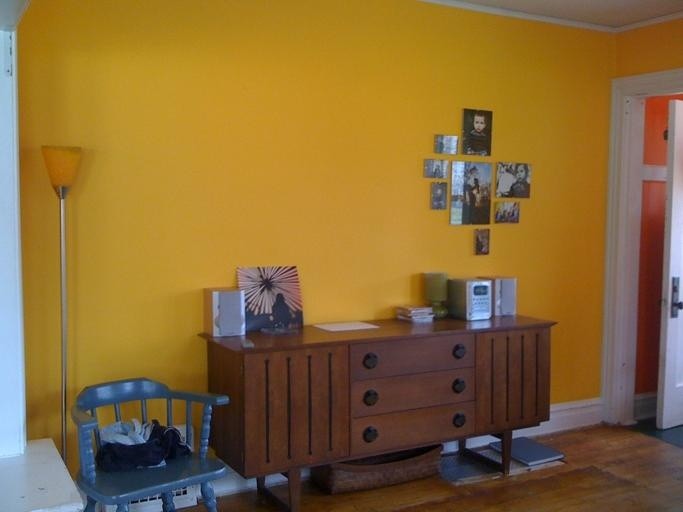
[508,163,530,196]
[468,111,488,156]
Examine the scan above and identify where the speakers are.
[494,278,517,316]
[212,290,246,337]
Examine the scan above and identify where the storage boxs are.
[311,443,445,495]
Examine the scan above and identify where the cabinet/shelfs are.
[197,313,560,511]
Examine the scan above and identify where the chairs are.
[71,377,230,511]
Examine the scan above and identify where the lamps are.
[423,272,448,318]
[40,144,83,463]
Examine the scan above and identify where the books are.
[396,305,434,323]
[438,453,503,488]
[478,436,566,475]
